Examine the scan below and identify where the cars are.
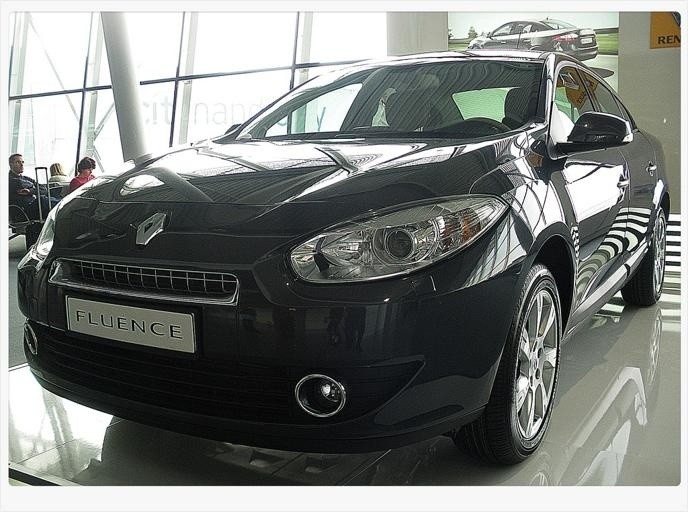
[467,19,600,62]
[18,50,671,466]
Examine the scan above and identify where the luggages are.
[25,167,52,253]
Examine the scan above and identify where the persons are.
[9,153,63,223]
[48,163,71,182]
[68,157,96,195]
